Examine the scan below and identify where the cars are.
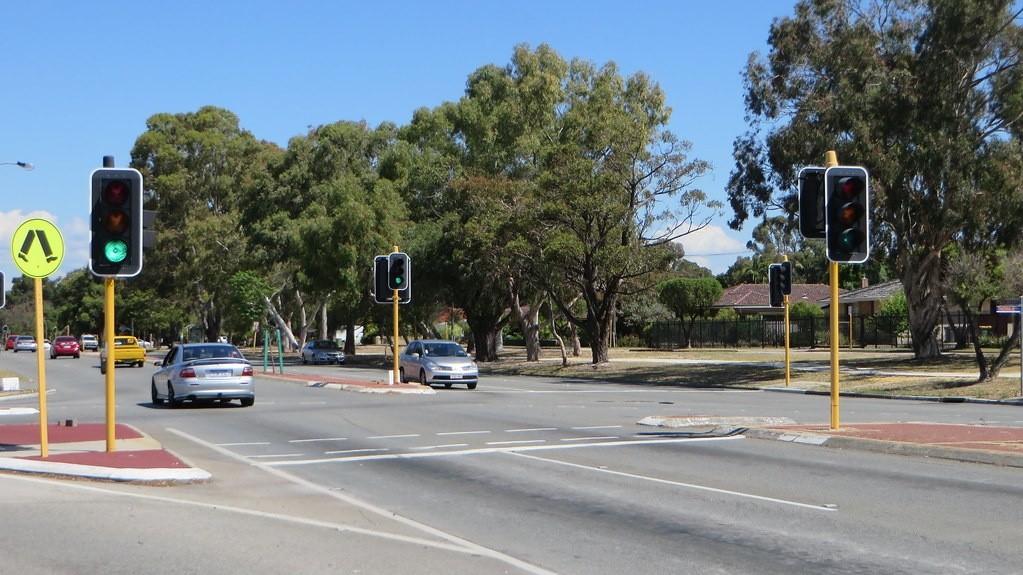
[398,338,480,389]
[138,338,151,348]
[99,335,147,366]
[14,335,37,353]
[79,334,98,352]
[150,343,255,409]
[49,335,81,359]
[44,339,50,349]
[301,339,345,366]
[5,335,21,351]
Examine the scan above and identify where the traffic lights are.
[777,261,792,294]
[824,165,870,264]
[142,208,159,248]
[387,252,408,290]
[88,167,144,278]
